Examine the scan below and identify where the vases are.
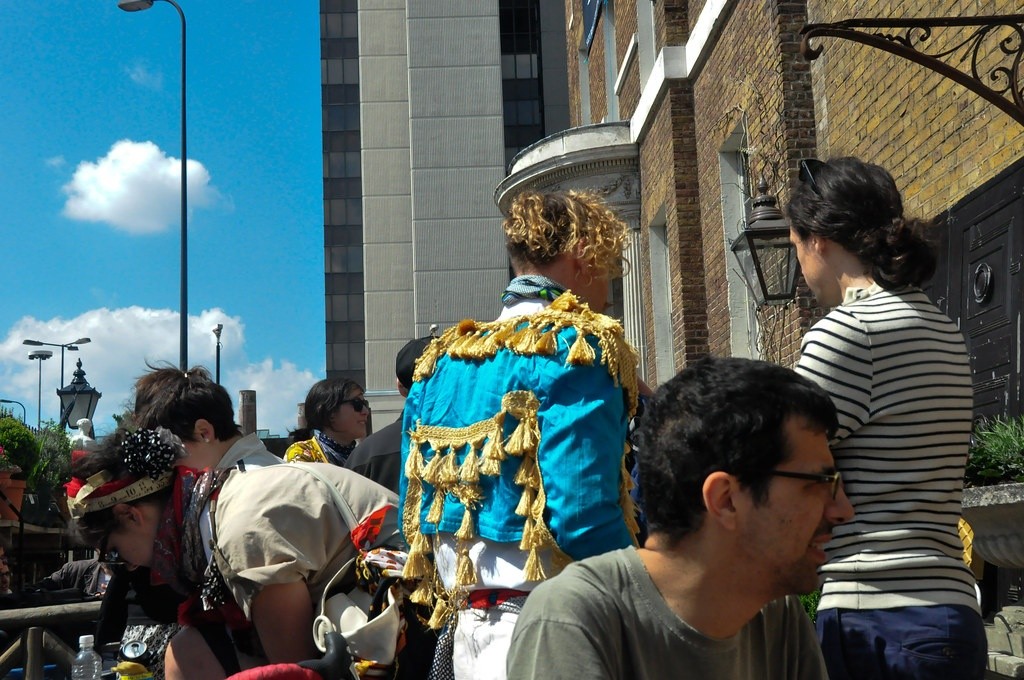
[0,472,11,520]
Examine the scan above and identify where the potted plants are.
[0,417,41,520]
[962,414,1024,570]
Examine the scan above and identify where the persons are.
[343,336,446,495]
[28,368,452,680]
[397,190,653,680]
[785,158,989,680]
[0,557,13,654]
[283,377,369,468]
[506,357,854,680]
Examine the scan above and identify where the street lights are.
[117,0,189,372]
[0,399,27,424]
[22,337,92,433]
[28,350,53,427]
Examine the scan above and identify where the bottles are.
[71,635,102,680]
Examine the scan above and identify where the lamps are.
[732,170,799,311]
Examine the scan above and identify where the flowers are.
[0,446,13,472]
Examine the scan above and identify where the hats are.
[395,336,439,388]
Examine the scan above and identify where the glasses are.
[770,470,841,501]
[0,571,10,577]
[799,157,826,199]
[97,502,139,566]
[0,556,8,564]
[342,397,369,412]
